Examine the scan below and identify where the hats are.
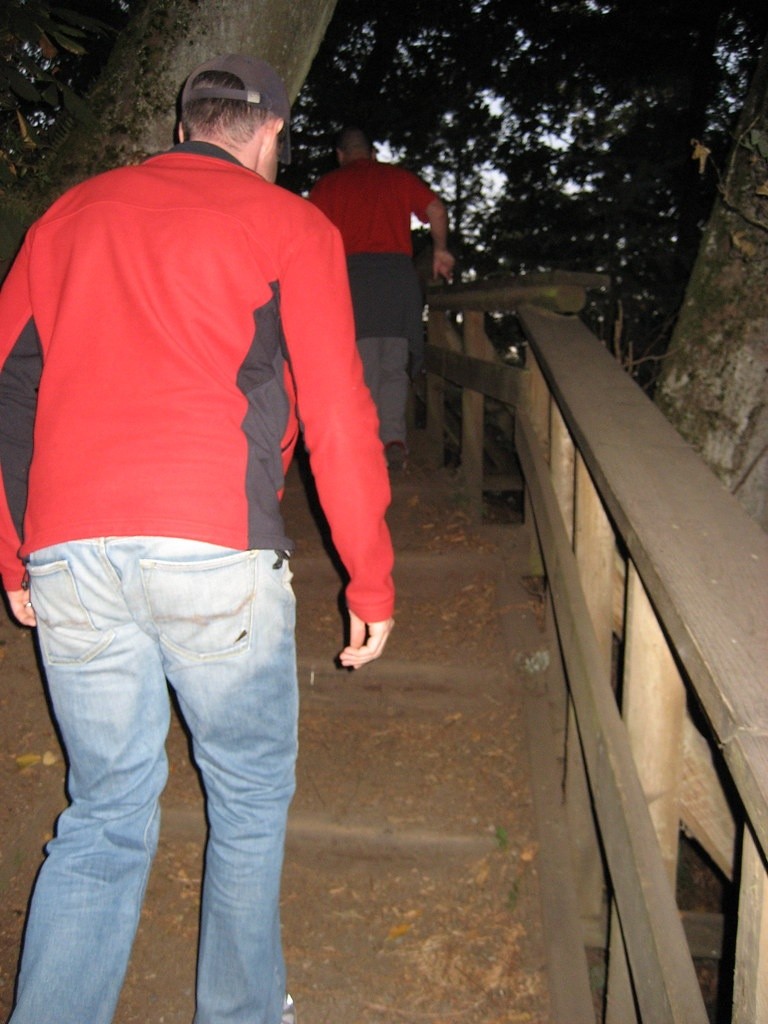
[182,55,292,166]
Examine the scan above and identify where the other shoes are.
[387,449,408,482]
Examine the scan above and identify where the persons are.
[1,52,397,1024]
[308,129,455,475]
